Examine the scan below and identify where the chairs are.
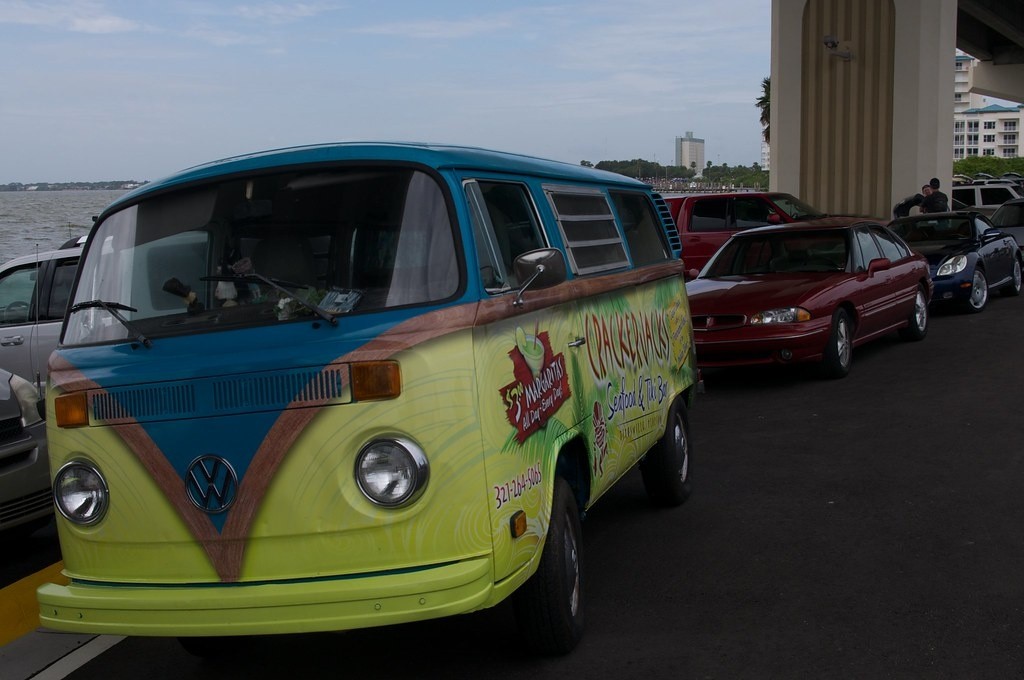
[783,248,808,270]
[956,221,971,238]
[915,225,936,242]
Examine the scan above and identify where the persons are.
[909,177,948,215]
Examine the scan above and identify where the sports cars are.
[886,210,1023,314]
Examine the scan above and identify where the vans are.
[37,140,704,657]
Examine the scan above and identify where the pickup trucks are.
[663,192,892,277]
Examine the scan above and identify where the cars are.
[953,172,1024,283]
[685,217,934,378]
[0,367,55,530]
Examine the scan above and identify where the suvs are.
[0,230,263,396]
